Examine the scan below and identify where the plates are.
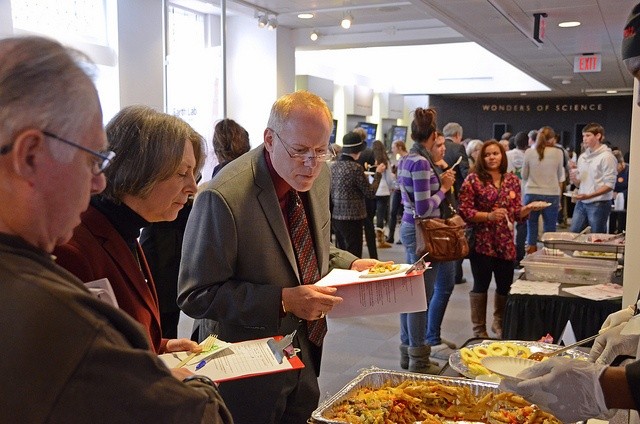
[482,355,549,384]
[363,170,380,175]
[359,263,409,277]
[448,339,594,383]
[562,190,582,199]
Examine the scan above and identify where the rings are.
[320,311,325,318]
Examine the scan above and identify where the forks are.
[368,164,380,168]
[172,333,220,371]
[450,155,463,172]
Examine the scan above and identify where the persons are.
[385,141,407,242]
[569,124,617,233]
[528,129,538,145]
[553,133,568,227]
[53,105,203,354]
[212,119,250,180]
[397,107,456,375]
[522,127,565,254]
[566,145,573,159]
[0,37,234,424]
[457,140,548,338]
[466,139,484,167]
[505,132,531,269]
[352,128,377,259]
[443,123,469,283]
[500,323,640,423]
[597,299,640,334]
[501,132,514,138]
[373,140,395,248]
[330,133,386,259]
[609,150,629,232]
[498,139,510,150]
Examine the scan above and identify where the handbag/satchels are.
[463,173,504,248]
[402,152,469,261]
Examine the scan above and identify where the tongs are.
[573,225,593,245]
[602,230,627,244]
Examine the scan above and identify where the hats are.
[342,132,366,153]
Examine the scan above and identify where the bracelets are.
[258,275,304,333]
[179,374,216,385]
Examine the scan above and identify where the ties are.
[287,189,327,346]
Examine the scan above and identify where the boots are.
[470,291,489,339]
[492,290,505,336]
[400,345,440,370]
[409,347,441,375]
[375,227,392,248]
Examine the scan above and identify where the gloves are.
[599,305,635,334]
[588,322,640,365]
[498,357,618,423]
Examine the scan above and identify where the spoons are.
[525,331,602,361]
[406,252,430,272]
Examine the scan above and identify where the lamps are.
[268,18,279,33]
[257,14,267,28]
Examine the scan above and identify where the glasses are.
[0,131,116,175]
[271,129,337,161]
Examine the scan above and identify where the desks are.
[502,274,624,346]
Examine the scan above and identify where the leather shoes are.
[430,343,456,360]
[440,339,457,349]
